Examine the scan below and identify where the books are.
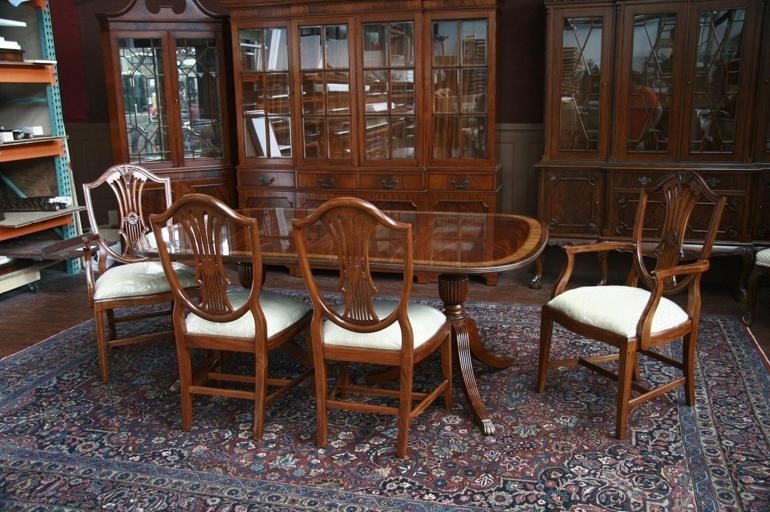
[1,36,21,50]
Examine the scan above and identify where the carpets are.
[0,284,770,511]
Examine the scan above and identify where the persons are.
[628,69,664,152]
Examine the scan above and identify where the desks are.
[136,204,548,436]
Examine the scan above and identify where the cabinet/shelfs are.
[534,2,765,326]
[229,3,500,285]
[0,0,82,296]
[106,2,234,236]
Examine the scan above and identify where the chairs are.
[536,168,726,441]
[290,195,454,460]
[148,195,314,439]
[81,164,196,380]
